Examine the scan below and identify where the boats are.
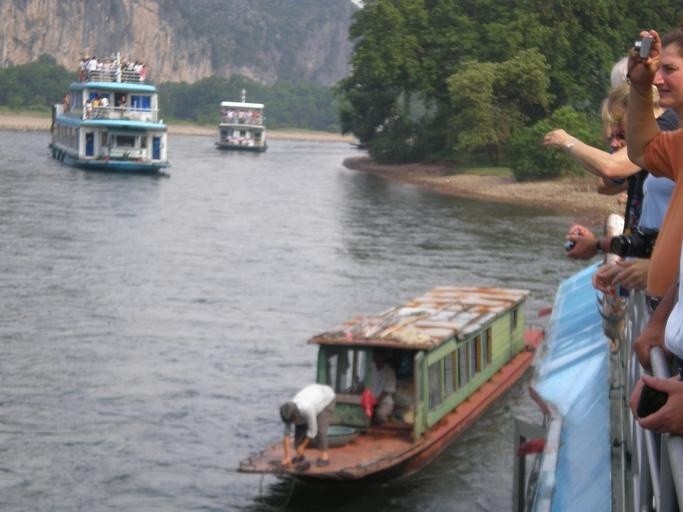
[48,51,167,173]
[234,284,549,489]
[215,87,268,152]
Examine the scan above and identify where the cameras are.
[564,229,581,251]
[610,224,659,258]
[634,34,655,59]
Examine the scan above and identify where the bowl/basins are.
[310,425,359,448]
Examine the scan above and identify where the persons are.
[77,56,147,82]
[85,95,127,115]
[279,384,336,467]
[362,353,397,426]
[219,107,262,146]
[63,93,69,113]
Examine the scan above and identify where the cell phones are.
[638,386,669,419]
